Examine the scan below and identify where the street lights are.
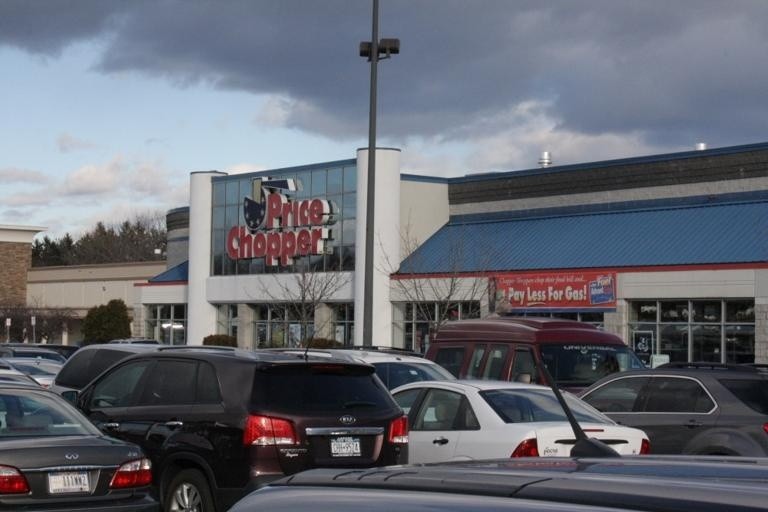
[357,37,405,351]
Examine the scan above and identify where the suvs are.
[65,344,410,511]
[582,360,768,458]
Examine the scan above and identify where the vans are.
[424,318,647,397]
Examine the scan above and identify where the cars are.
[2,382,161,511]
[281,345,456,403]
[388,379,652,467]
[50,342,238,405]
[4,336,79,394]
[236,458,767,511]
